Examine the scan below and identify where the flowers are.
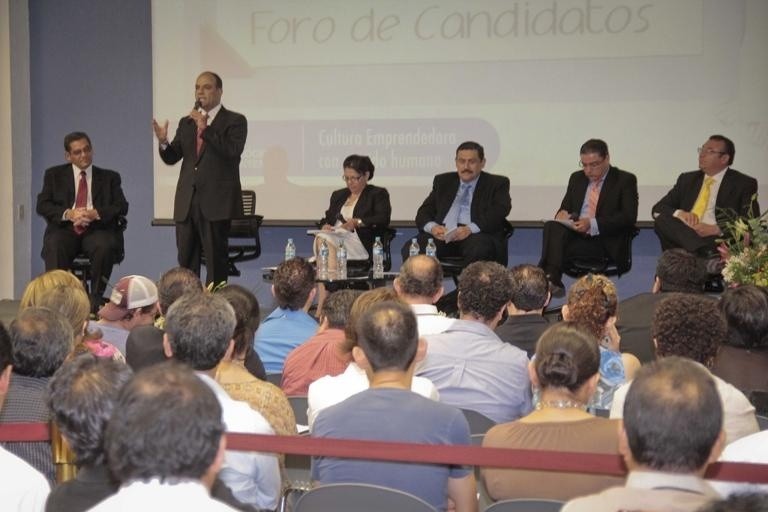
[718,205,768,288]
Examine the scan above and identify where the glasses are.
[579,160,606,168]
[342,175,363,182]
[697,147,725,156]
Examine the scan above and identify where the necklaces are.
[538,402,586,411]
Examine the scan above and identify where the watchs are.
[356,218,362,227]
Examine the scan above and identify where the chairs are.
[562,222,641,279]
[266,369,612,512]
[305,222,397,270]
[430,217,516,286]
[193,188,266,286]
[57,215,130,315]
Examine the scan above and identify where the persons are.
[38,132,129,320]
[539,140,638,296]
[530,274,638,417]
[711,287,768,415]
[43,358,255,512]
[652,136,762,294]
[41,287,90,348]
[280,290,363,395]
[414,260,530,428]
[700,490,768,512]
[311,302,478,511]
[479,324,629,505]
[615,248,719,368]
[609,296,760,450]
[91,364,242,510]
[20,270,128,367]
[95,274,159,360]
[703,428,768,502]
[395,254,456,339]
[312,155,389,323]
[0,323,50,512]
[561,358,728,512]
[400,142,513,274]
[495,263,550,361]
[162,295,280,512]
[210,285,298,474]
[253,256,322,375]
[0,304,74,489]
[125,267,264,384]
[307,286,439,433]
[151,72,248,292]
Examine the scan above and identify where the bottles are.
[317,239,328,280]
[336,239,347,280]
[426,239,436,257]
[372,237,384,280]
[284,238,295,260]
[409,238,420,257]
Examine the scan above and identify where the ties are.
[444,184,470,243]
[691,176,712,222]
[196,112,212,158]
[73,171,88,235]
[588,181,600,218]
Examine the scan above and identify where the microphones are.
[187,101,200,124]
[336,213,354,233]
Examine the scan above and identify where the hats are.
[98,275,158,321]
